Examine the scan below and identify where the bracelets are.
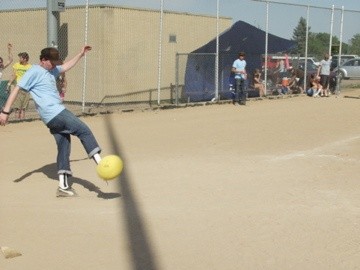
[2,110,10,114]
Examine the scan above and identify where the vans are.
[272,56,319,72]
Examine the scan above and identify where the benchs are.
[246,88,256,98]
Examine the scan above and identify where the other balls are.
[96,154,125,180]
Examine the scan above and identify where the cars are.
[329,55,360,80]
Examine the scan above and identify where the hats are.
[239,52,245,56]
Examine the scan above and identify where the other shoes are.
[58,186,77,197]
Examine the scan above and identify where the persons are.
[0,46,101,197]
[330,63,338,95]
[258,54,304,99]
[0,44,12,83]
[4,52,31,120]
[307,74,322,98]
[316,56,331,97]
[254,71,264,97]
[231,51,249,106]
